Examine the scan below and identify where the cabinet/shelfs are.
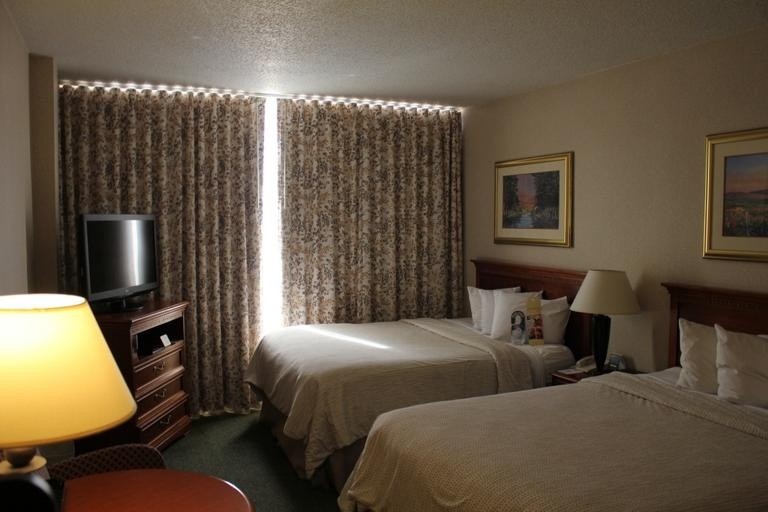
[88,301,191,451]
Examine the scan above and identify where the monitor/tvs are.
[75,213,162,315]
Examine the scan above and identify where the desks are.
[63,466,251,512]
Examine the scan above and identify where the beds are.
[337,280,767,511]
[242,259,589,494]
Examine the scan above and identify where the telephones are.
[576,355,597,373]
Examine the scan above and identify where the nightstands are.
[548,364,647,385]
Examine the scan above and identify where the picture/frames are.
[492,150,575,248]
[703,126,768,262]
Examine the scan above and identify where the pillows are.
[715,322,768,407]
[674,317,717,394]
[463,283,570,347]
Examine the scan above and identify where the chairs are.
[47,443,165,493]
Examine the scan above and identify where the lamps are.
[569,269,644,365]
[0,292,138,512]
